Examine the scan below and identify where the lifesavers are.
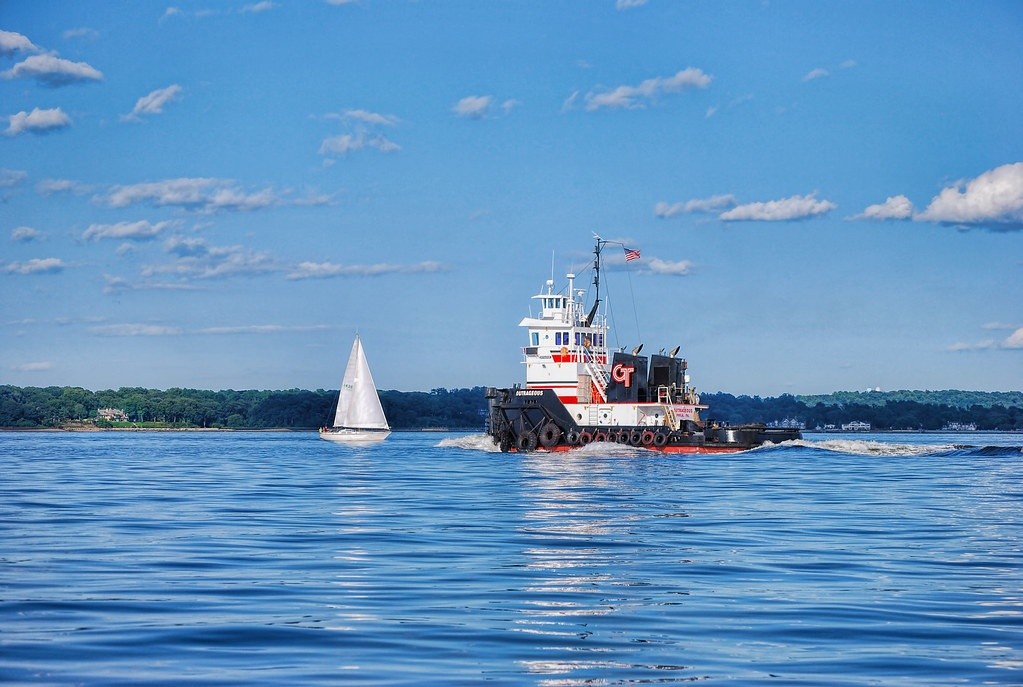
[642,431,654,446]
[538,421,562,448]
[494,430,512,453]
[580,430,592,446]
[515,430,538,453]
[654,431,668,448]
[592,432,606,445]
[561,347,568,357]
[618,430,630,446]
[566,429,580,446]
[630,431,642,447]
[607,432,618,444]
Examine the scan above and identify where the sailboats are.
[319,330,389,441]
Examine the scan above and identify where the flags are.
[624,248,640,261]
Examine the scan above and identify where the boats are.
[490,232,802,454]
